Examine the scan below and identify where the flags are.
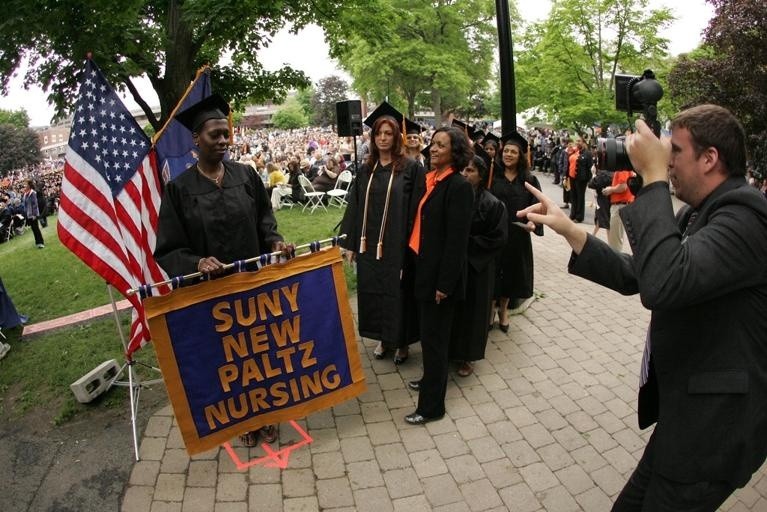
[56,54,213,357]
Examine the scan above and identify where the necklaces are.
[197,159,223,183]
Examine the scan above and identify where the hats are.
[363,101,427,148]
[452,118,531,189]
[173,92,233,144]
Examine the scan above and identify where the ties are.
[639,209,698,388]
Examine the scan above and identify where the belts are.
[610,201,631,205]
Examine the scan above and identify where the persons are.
[0,168,63,245]
[20,179,44,248]
[338,101,545,423]
[517,106,766,511]
[226,91,633,224]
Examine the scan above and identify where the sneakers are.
[259,426,276,443]
[238,430,256,446]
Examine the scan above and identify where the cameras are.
[597,67,665,196]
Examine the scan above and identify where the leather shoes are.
[409,380,422,389]
[394,346,409,364]
[404,412,444,424]
[459,360,475,375]
[373,343,388,358]
[487,308,509,333]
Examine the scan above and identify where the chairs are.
[327,170,352,209]
[277,183,303,211]
[4,220,13,241]
[298,175,327,214]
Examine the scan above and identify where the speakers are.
[336,99,363,137]
